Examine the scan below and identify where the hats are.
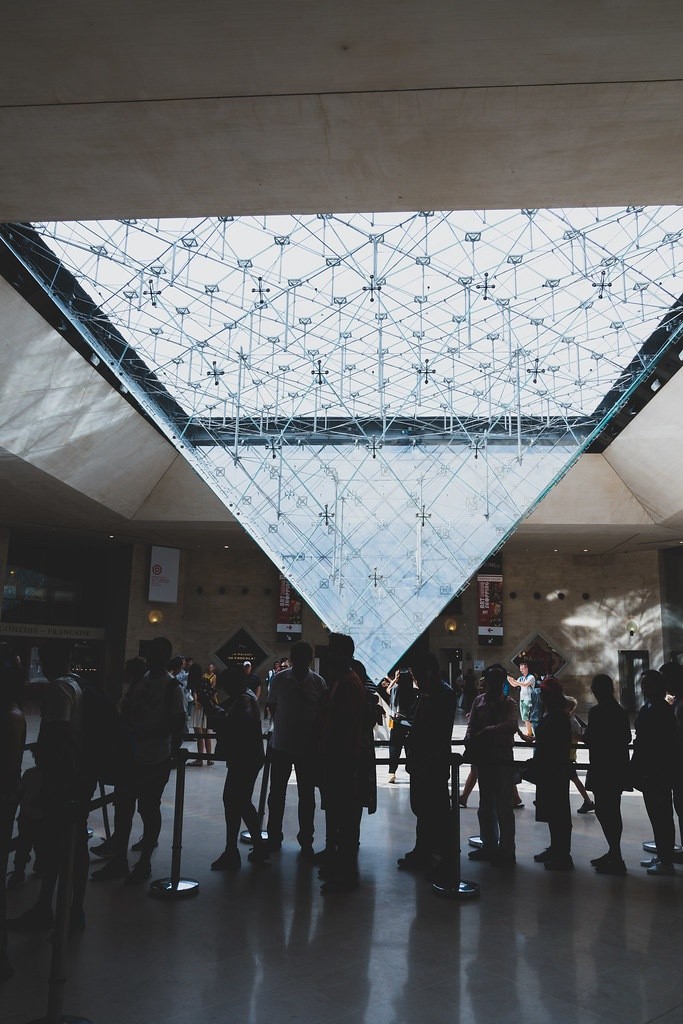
[243,662,252,666]
[537,676,562,695]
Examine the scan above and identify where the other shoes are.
[647,862,676,876]
[208,762,215,765]
[90,836,127,859]
[597,860,628,873]
[300,846,314,861]
[131,837,160,850]
[589,851,607,866]
[248,846,271,862]
[314,850,332,862]
[263,842,283,851]
[317,863,360,894]
[187,760,204,767]
[398,858,406,866]
[10,870,26,884]
[534,845,550,864]
[470,845,493,861]
[211,848,243,871]
[389,774,396,784]
[124,859,152,882]
[578,801,595,813]
[641,856,656,866]
[492,850,515,867]
[8,907,53,931]
[546,856,574,871]
[93,862,122,880]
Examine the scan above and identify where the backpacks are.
[54,675,123,785]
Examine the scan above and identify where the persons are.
[0,631,375,930]
[381,651,683,880]
